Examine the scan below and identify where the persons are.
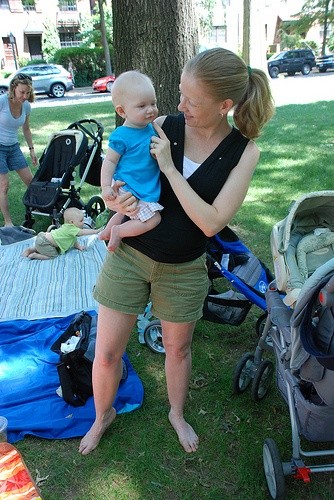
[22,207,102,260]
[0,74,39,227]
[79,47,273,457]
[98,71,162,252]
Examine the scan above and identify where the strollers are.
[232,190,334,500]
[143,225,294,355]
[20,118,106,234]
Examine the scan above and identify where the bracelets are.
[29,147,34,150]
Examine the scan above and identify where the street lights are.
[9,32,18,71]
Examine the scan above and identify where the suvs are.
[0,64,75,98]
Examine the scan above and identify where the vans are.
[267,49,317,79]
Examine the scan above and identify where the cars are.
[92,76,116,93]
[317,55,334,73]
